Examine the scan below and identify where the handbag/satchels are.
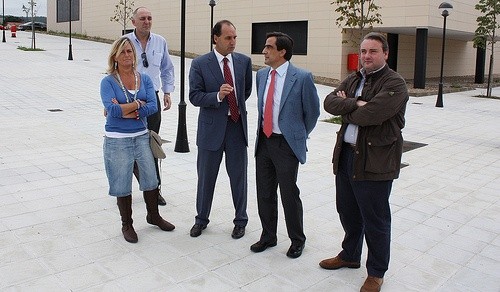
[149,130,171,159]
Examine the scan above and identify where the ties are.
[223,58,240,123]
[263,70,277,138]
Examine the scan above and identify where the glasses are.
[141,52,149,67]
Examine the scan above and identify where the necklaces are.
[115,70,138,104]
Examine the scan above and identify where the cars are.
[19,21,47,31]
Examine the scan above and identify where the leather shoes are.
[250,239,277,252]
[286,242,305,258]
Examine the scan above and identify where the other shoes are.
[158,194,167,206]
[360,276,384,292]
[319,253,361,270]
[231,225,245,238]
[190,223,207,237]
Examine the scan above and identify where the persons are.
[123,6,175,206]
[250,32,321,258]
[100,36,175,242]
[319,32,409,292]
[189,20,253,237]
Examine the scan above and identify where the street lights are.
[435,2,454,107]
[208,0,216,52]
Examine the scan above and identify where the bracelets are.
[135,110,139,120]
[135,99,142,109]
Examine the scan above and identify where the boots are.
[117,195,138,243]
[143,187,175,231]
